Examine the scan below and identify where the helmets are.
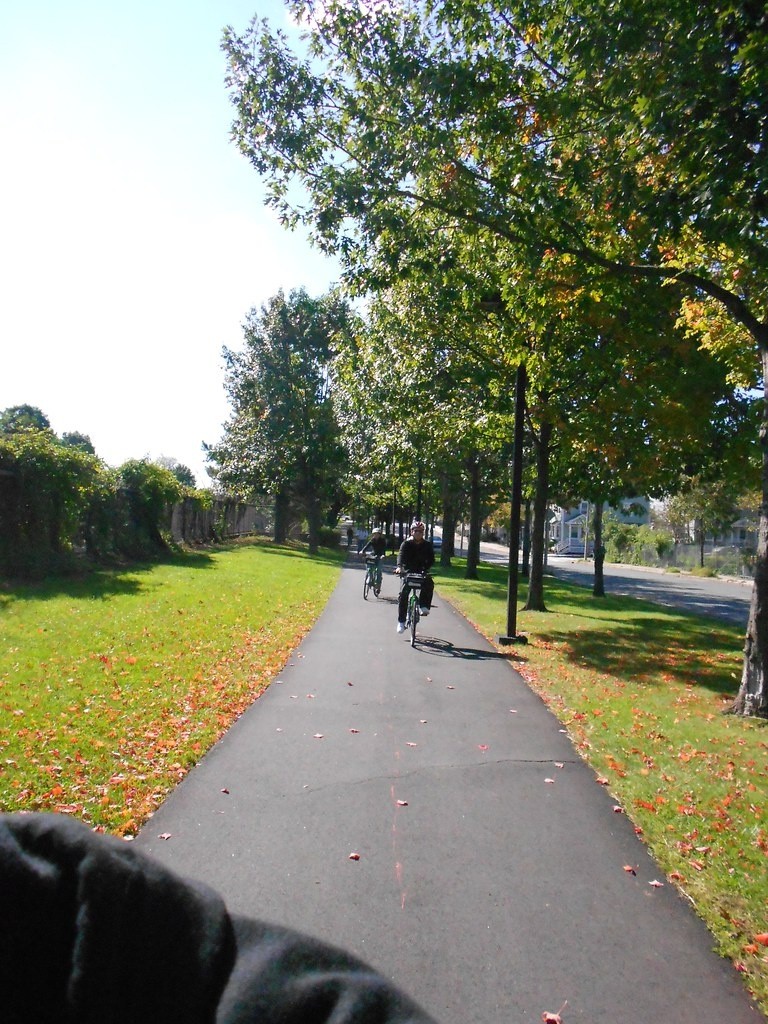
[410,520,424,530]
[372,528,381,534]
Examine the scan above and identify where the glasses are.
[415,530,422,533]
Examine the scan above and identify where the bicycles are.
[393,570,437,647]
[360,552,388,600]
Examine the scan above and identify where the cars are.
[344,515,350,521]
[428,536,442,547]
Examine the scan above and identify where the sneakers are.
[416,606,429,615]
[397,621,406,633]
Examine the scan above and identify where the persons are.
[347,526,353,547]
[358,527,387,594]
[356,524,369,555]
[0,811,437,1024]
[395,522,435,633]
